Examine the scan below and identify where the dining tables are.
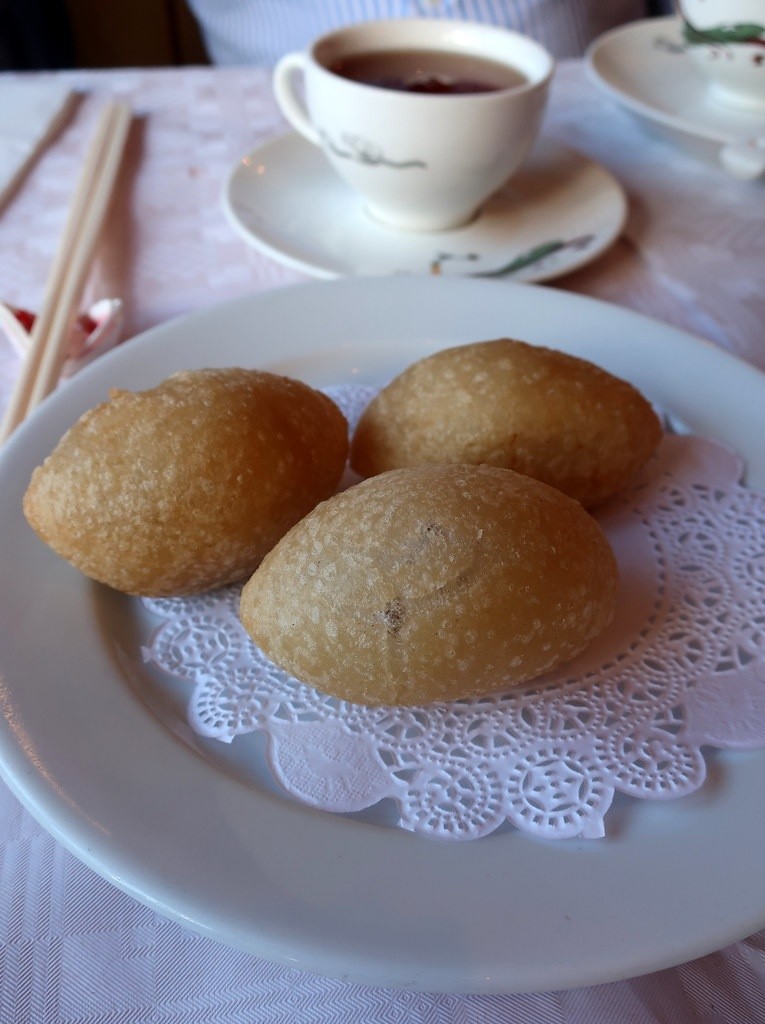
[0,60,765,1024]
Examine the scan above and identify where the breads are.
[239,463,622,706]
[21,367,350,597]
[348,337,663,508]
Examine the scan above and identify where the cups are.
[674,1,765,110]
[270,19,557,232]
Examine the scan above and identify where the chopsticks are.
[1,92,131,447]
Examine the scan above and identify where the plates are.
[223,127,629,285]
[584,15,764,162]
[1,278,764,996]
